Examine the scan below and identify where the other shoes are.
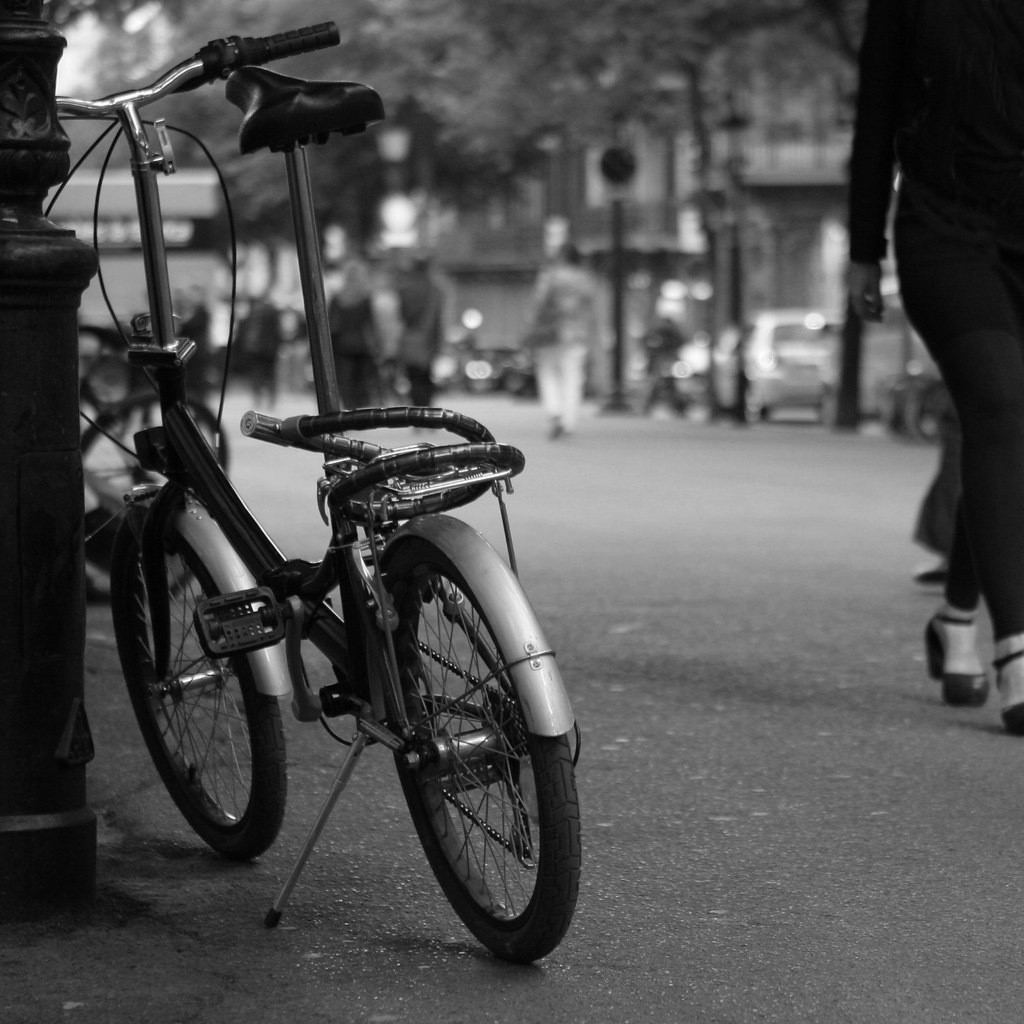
[548,416,565,439]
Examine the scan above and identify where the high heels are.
[993,651,1024,735]
[925,614,989,703]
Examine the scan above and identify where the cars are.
[711,302,959,441]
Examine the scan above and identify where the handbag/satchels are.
[400,332,430,365]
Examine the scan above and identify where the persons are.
[179,284,210,399]
[235,290,278,407]
[640,318,688,418]
[327,261,378,411]
[912,391,963,584]
[395,254,441,407]
[844,0,1024,735]
[529,244,590,437]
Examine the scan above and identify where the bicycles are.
[49,18,586,964]
